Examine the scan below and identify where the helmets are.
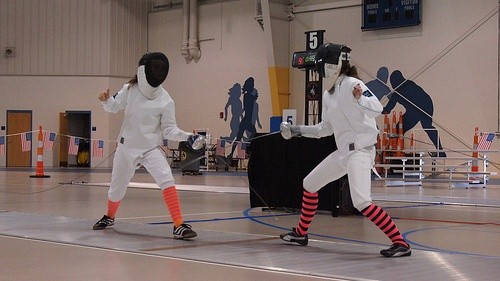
[314,43,352,92]
[137,52,169,100]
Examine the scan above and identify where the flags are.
[44,132,57,150]
[217,139,225,155]
[477,132,495,150]
[69,136,79,155]
[238,143,246,158]
[0,136,5,154]
[21,132,31,151]
[162,140,168,154]
[94,140,104,158]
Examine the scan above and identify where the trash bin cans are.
[77,142,89,163]
[179,142,201,176]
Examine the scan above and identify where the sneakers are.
[173,223,197,239]
[280,227,308,245]
[380,243,411,257]
[93,215,115,229]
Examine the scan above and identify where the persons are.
[280,43,411,257]
[93,52,200,238]
